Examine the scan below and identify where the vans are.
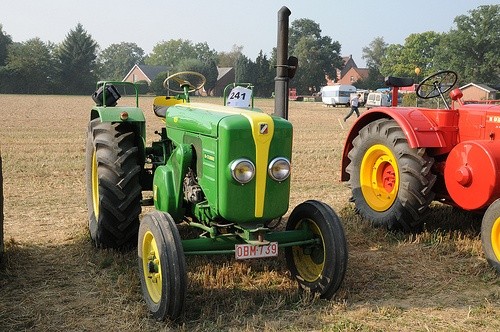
[365,92,390,110]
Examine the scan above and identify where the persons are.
[344,94,361,122]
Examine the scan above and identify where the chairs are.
[385,75,413,106]
[152,96,187,124]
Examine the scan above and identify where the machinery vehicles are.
[339,68,499,274]
[84,5,348,323]
